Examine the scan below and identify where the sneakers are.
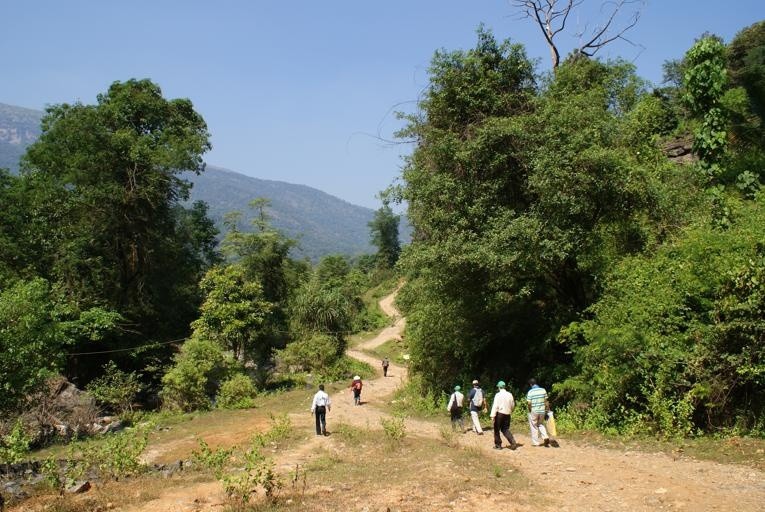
[478,433,484,435]
[317,431,326,436]
[545,438,549,447]
[492,445,517,450]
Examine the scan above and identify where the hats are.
[354,376,361,380]
[496,380,506,387]
[385,355,388,359]
[454,385,461,390]
[472,380,479,384]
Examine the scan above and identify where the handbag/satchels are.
[546,410,556,436]
[452,398,458,412]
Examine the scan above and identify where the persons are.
[489,380,518,450]
[467,380,488,434]
[381,356,390,377]
[350,374,362,406]
[526,378,550,446]
[310,384,332,436]
[446,385,466,434]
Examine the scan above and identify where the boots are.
[354,400,361,405]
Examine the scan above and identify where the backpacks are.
[474,389,483,407]
[356,383,361,390]
[384,361,387,366]
